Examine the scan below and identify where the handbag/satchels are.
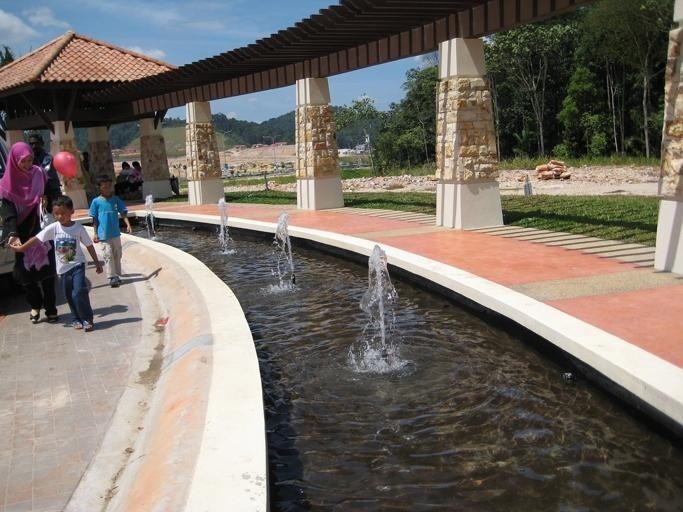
[40,213,55,230]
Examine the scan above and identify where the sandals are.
[74,321,94,332]
[30,309,40,324]
[48,315,57,323]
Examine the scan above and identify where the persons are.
[0,134,133,332]
[114,161,144,195]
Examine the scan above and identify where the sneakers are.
[110,276,122,287]
